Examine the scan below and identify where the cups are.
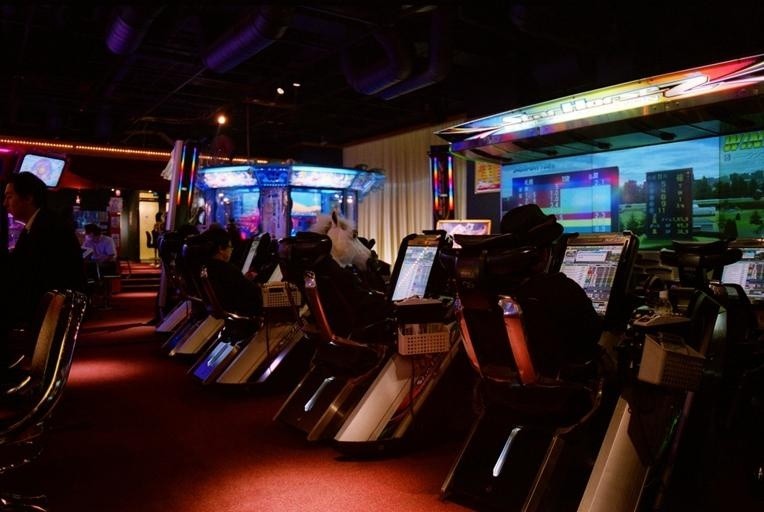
[225,244,235,250]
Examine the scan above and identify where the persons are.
[204,229,261,315]
[1,171,87,319]
[461,203,602,381]
[81,223,118,278]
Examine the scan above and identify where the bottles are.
[454,203,556,252]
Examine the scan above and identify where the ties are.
[385,234,441,300]
[436,219,492,256]
[712,239,764,308]
[17,152,65,189]
[553,230,639,332]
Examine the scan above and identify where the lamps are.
[175,285,262,389]
[0,289,87,512]
[439,230,605,512]
[271,234,387,443]
